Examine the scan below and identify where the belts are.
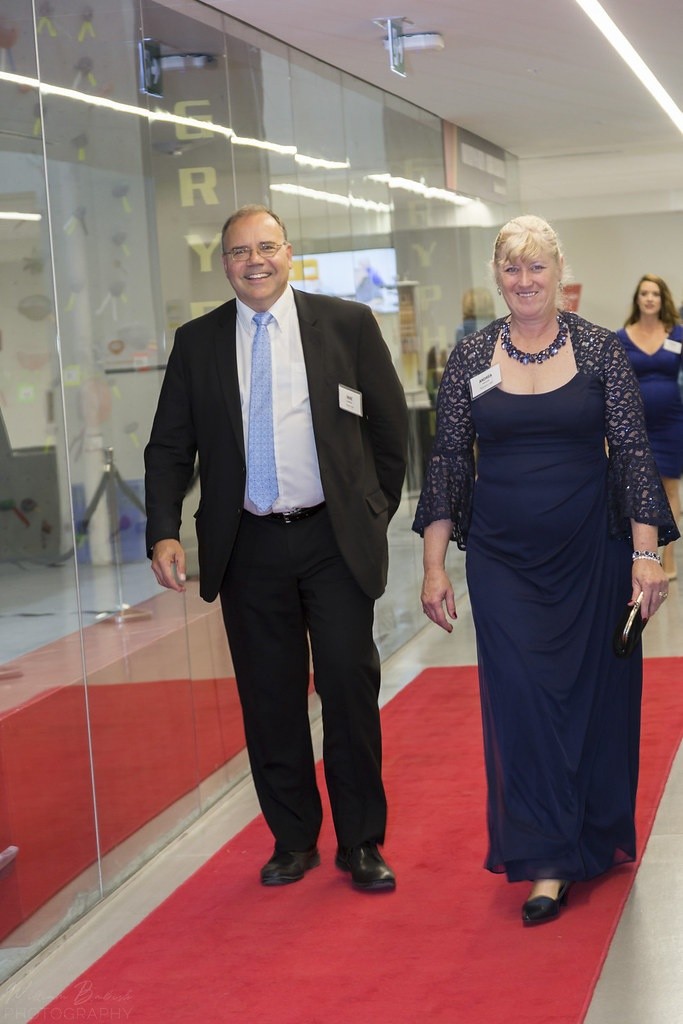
[242,501,325,523]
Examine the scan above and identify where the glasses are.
[224,241,284,261]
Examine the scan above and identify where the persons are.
[144,205,409,891]
[411,215,681,927]
[613,274,683,582]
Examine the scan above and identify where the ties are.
[246,313,280,510]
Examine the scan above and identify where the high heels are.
[522,879,576,923]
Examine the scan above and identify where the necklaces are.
[500,315,568,365]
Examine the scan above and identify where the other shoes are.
[665,559,678,581]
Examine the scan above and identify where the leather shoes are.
[260,844,320,884]
[335,844,395,890]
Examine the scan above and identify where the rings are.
[662,592,667,598]
[659,592,663,596]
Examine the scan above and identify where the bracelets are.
[632,550,661,563]
